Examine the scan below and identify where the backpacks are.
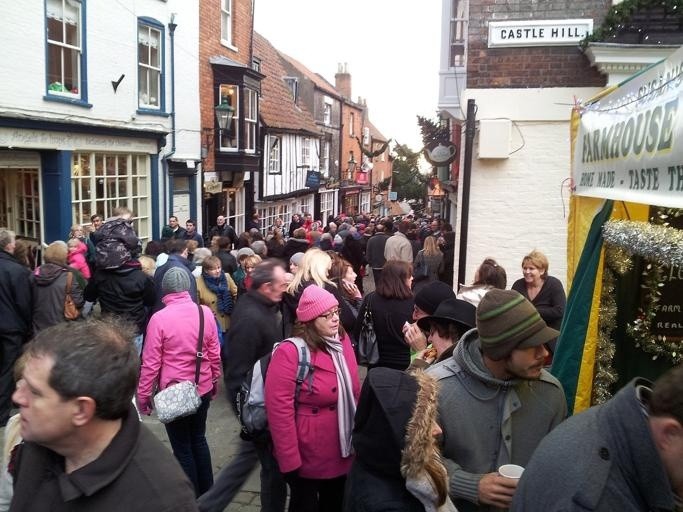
[235,351,273,440]
[153,379,203,424]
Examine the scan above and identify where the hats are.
[296,284,339,322]
[474,288,561,362]
[250,240,265,254]
[237,247,255,264]
[162,266,191,294]
[416,298,477,337]
[414,280,456,316]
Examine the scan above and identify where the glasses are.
[429,324,443,336]
[318,308,341,319]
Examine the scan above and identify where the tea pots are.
[425,142,457,162]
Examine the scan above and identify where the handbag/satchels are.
[63,295,80,320]
[357,320,379,366]
[413,264,428,279]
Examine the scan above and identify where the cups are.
[498,463,526,485]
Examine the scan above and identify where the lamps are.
[208,94,235,134]
[342,156,357,174]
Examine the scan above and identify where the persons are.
[1,212,569,511]
[509,364,683,511]
